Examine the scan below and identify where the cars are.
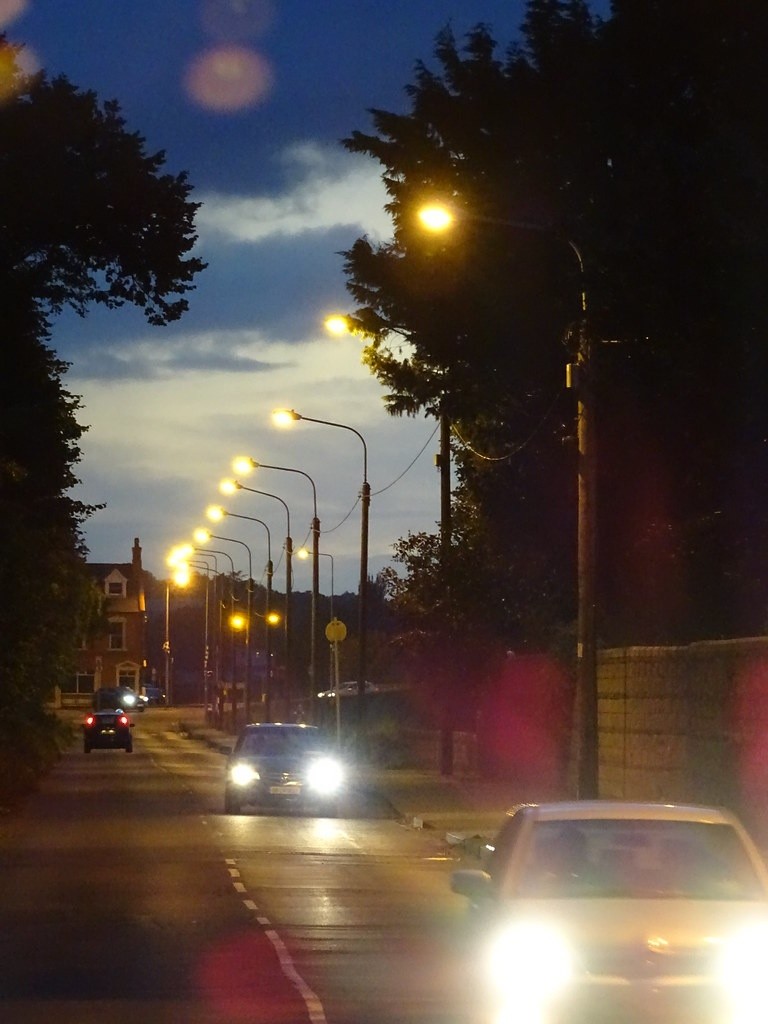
[317,680,381,703]
[219,721,343,818]
[82,711,135,753]
[146,687,167,707]
[447,797,767,1024]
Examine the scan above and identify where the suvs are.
[94,686,147,712]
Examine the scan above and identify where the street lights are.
[327,311,458,779]
[218,478,296,722]
[233,458,323,757]
[416,205,601,807]
[203,502,276,722]
[165,529,256,727]
[273,405,370,771]
